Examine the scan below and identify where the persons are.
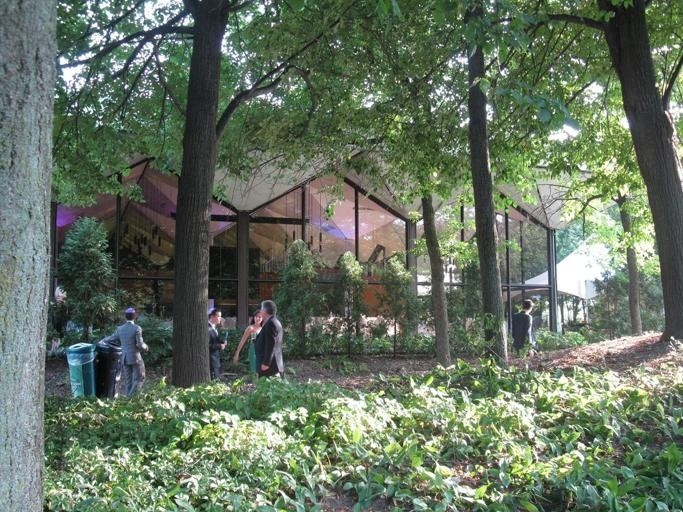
[253,299,284,381]
[207,307,227,383]
[510,298,535,355]
[231,308,263,377]
[102,307,149,397]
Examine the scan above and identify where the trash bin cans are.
[65,336,123,401]
